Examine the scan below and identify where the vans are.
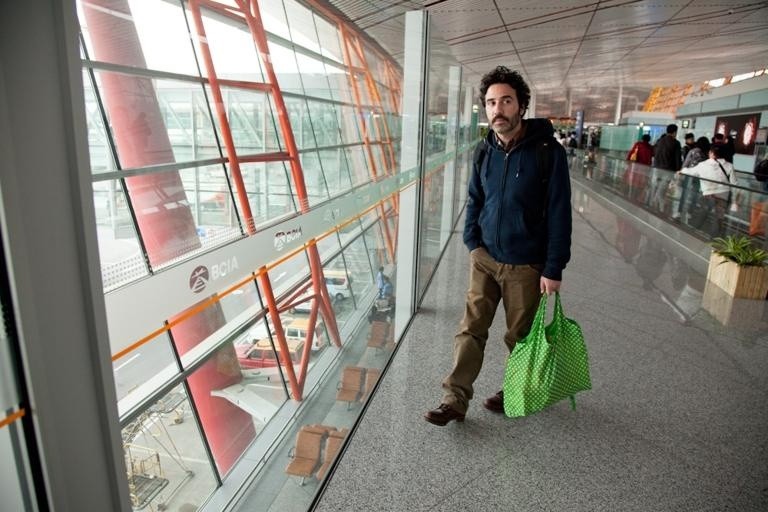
[304,269,354,300]
[288,291,337,315]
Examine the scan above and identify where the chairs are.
[283,319,394,481]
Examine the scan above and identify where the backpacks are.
[568,136,577,148]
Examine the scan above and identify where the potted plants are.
[705,233,768,300]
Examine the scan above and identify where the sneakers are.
[424,404,465,426]
[483,391,504,413]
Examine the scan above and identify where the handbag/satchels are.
[503,317,592,418]
[630,153,637,161]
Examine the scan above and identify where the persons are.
[423,65,572,427]
[367,264,395,324]
[617,217,767,349]
[555,129,599,181]
[624,124,767,238]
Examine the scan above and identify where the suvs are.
[246,316,325,352]
[233,336,305,369]
